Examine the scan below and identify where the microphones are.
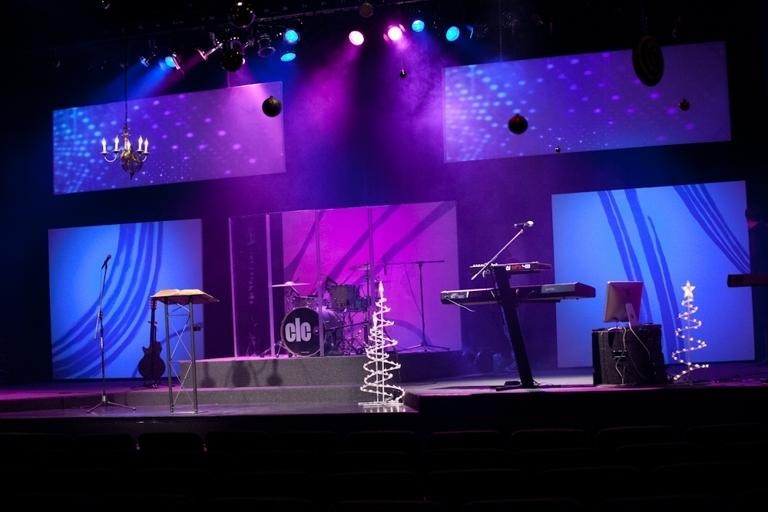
[383,256,387,276]
[101,255,111,269]
[514,221,533,229]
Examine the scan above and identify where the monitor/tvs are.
[604,281,644,326]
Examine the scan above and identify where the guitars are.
[138,298,165,379]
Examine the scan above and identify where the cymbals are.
[272,281,310,288]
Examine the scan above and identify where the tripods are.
[259,288,292,358]
[388,260,451,351]
[326,310,369,355]
[81,269,137,413]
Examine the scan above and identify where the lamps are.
[101,60,150,180]
[671,280,710,384]
[139,0,489,76]
[358,281,406,408]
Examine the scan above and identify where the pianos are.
[441,282,595,304]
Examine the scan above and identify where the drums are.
[327,285,359,310]
[280,305,344,356]
[360,296,381,311]
